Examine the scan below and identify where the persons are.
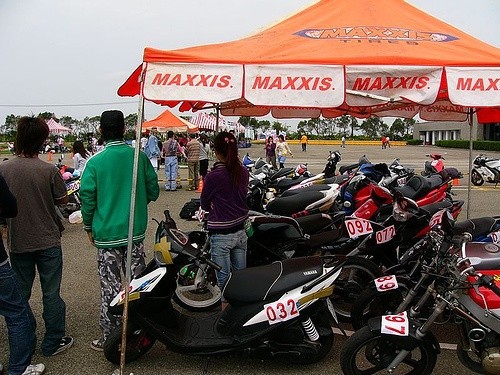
[178,133,210,191]
[132,136,137,148]
[73,141,92,171]
[341,136,346,148]
[299,134,308,152]
[382,135,386,149]
[140,134,148,148]
[8,141,15,155]
[162,130,188,191]
[266,136,278,169]
[0,173,45,375]
[144,127,161,172]
[386,136,391,148]
[276,135,294,169]
[201,131,249,312]
[80,110,160,351]
[0,117,74,357]
[45,136,64,153]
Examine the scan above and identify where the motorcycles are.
[470,153,500,189]
[101,153,500,375]
[57,154,81,218]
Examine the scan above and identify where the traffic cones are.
[194,175,203,193]
[46,152,53,161]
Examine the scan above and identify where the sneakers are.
[51,336,74,356]
[90,339,104,352]
[22,363,45,375]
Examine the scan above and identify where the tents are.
[135,109,198,133]
[117,0,499,375]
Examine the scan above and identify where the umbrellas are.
[47,118,72,133]
